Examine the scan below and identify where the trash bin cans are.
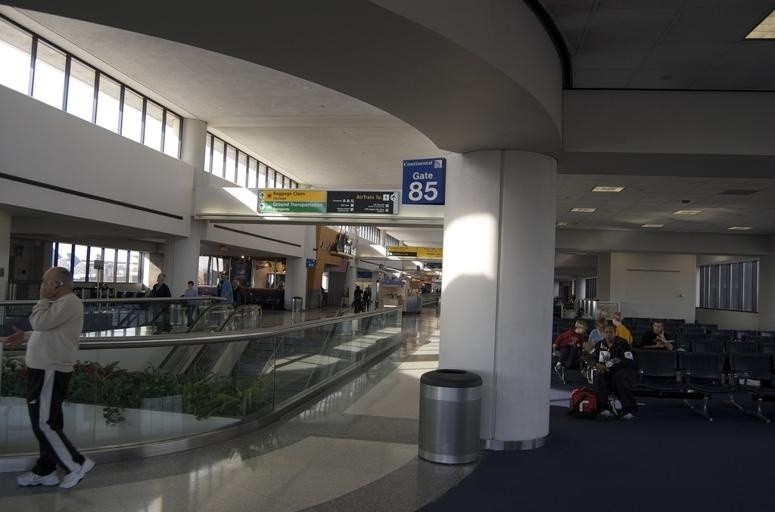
[292,297,302,311]
[418,369,483,464]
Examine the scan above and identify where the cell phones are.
[54,281,63,289]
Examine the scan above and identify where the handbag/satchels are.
[570,388,599,419]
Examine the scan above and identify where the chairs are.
[113,290,146,310]
[551,317,775,424]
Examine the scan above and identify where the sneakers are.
[621,413,637,422]
[600,410,616,420]
[59,455,96,489]
[16,469,59,487]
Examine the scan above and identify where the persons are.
[183,280,199,328]
[554,292,635,379]
[6,266,97,490]
[353,285,364,315]
[362,287,372,312]
[640,319,686,352]
[147,272,174,335]
[217,270,235,326]
[584,322,640,422]
